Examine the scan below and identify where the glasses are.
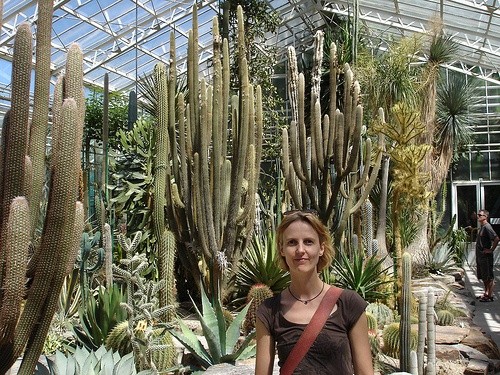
[478,214,486,217]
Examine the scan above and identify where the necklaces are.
[288,280,324,304]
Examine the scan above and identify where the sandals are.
[479,295,494,302]
[476,293,488,299]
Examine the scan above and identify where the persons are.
[475,209,499,302]
[255,211,373,375]
[467,210,478,242]
